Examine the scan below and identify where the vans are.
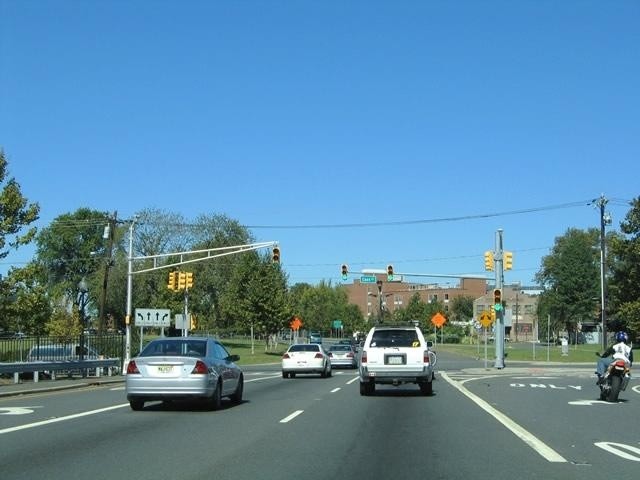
[27,344,100,361]
[310,333,322,344]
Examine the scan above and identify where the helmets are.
[615,332,628,343]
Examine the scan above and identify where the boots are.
[596,375,605,385]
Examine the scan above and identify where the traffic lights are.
[493,289,503,312]
[341,264,348,280]
[167,271,193,293]
[387,265,393,281]
[485,251,494,271]
[190,314,198,331]
[273,247,280,271]
[503,251,513,271]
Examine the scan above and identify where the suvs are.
[360,327,433,395]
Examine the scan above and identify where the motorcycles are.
[595,352,630,401]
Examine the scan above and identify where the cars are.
[281,344,331,378]
[327,333,366,369]
[126,337,243,411]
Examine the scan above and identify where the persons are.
[596,331,633,385]
